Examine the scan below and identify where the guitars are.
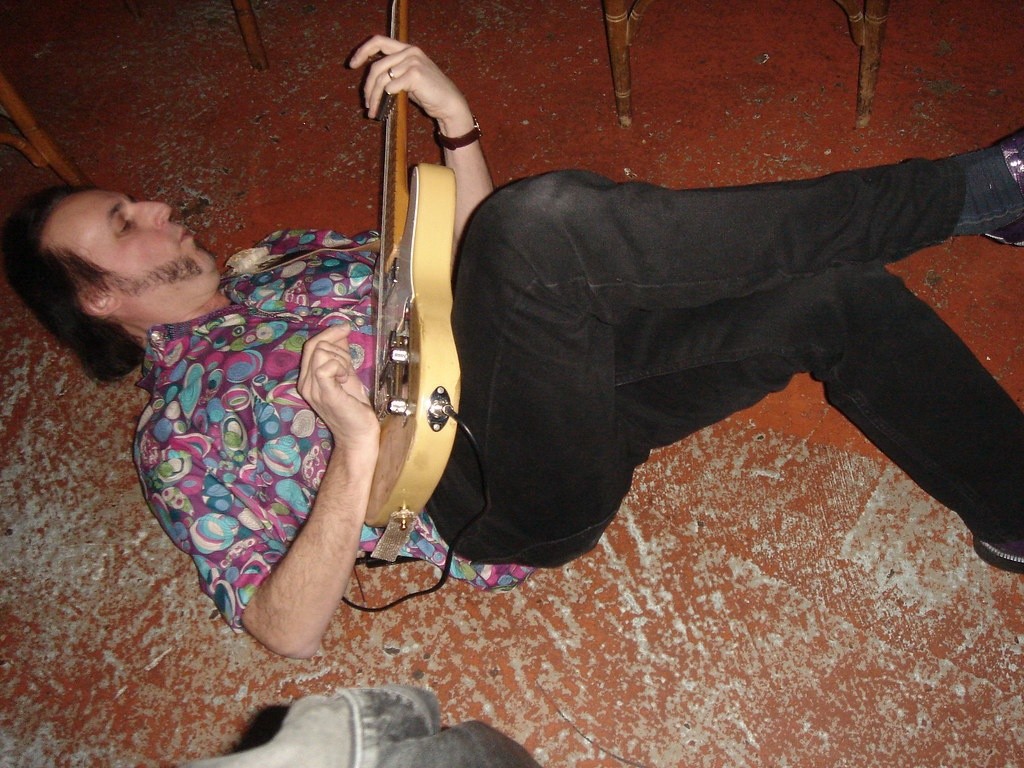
[361,0,462,530]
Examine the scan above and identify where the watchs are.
[438,113,483,150]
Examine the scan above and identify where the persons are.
[0,34,1023,658]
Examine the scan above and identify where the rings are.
[388,68,394,79]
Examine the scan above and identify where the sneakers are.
[973,535,1024,575]
[984,131,1024,247]
